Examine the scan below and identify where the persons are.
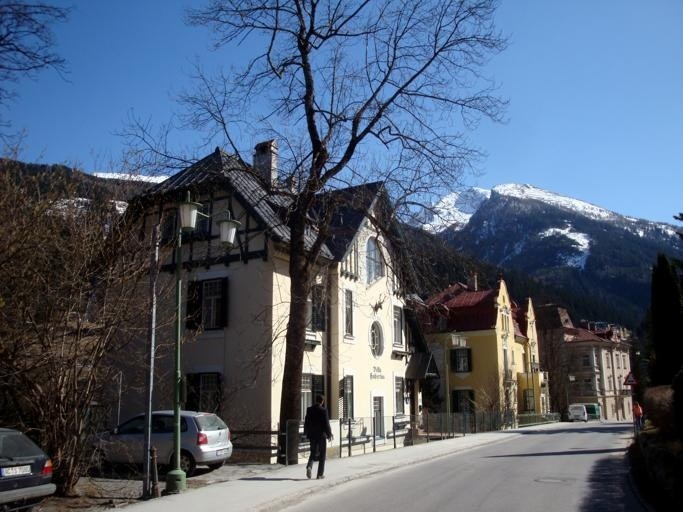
[632,401,642,430]
[304,394,332,478]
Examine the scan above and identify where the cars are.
[567,404,588,422]
[0,427,57,511]
[80,409,232,478]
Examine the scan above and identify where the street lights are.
[164,188,241,493]
[443,328,468,437]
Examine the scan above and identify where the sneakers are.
[317,475,325,479]
[307,467,312,479]
